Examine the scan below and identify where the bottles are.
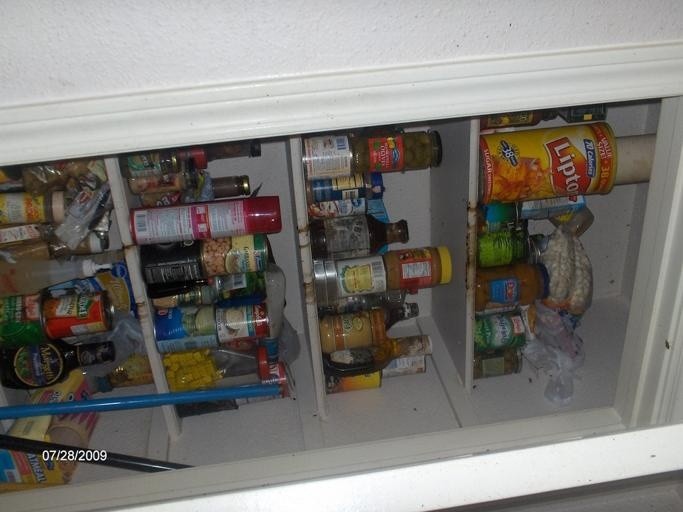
[0,259,111,296]
[160,345,269,392]
[0,337,115,389]
[128,169,184,194]
[48,225,104,257]
[116,148,208,177]
[473,265,552,380]
[0,191,66,228]
[85,374,112,396]
[480,107,557,133]
[180,175,250,203]
[299,124,454,394]
[0,222,48,247]
[204,142,261,162]
